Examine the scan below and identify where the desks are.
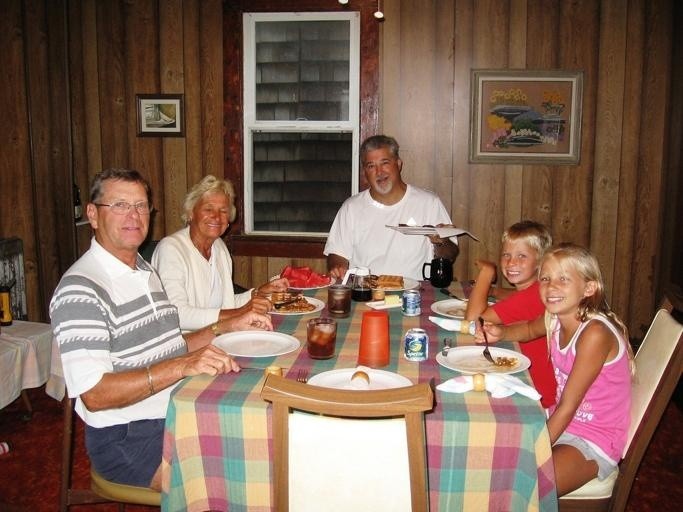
[0,320,53,415]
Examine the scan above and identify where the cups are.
[307,317,337,359]
[328,285,351,318]
[422,258,453,288]
[358,311,390,368]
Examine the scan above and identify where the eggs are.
[350,371,369,388]
[473,373,485,391]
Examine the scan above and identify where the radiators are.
[1,235,29,322]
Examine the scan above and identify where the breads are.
[272,293,292,304]
[377,275,404,288]
[385,295,399,304]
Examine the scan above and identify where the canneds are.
[402,289,422,316]
[403,329,429,361]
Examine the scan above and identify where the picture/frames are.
[469,69,583,164]
[135,93,186,138]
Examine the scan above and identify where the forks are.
[296,369,309,384]
[442,337,450,358]
[479,317,494,363]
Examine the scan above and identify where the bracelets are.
[146,365,154,395]
[253,286,259,296]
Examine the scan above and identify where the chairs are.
[628,279,683,357]
[58,384,161,511]
[557,297,683,510]
[260,374,435,512]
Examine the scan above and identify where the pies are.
[278,298,315,312]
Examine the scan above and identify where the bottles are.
[0,286,13,327]
[351,266,373,302]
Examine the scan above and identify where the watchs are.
[211,322,221,337]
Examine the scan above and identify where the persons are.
[473,241,633,498]
[151,175,291,336]
[49,167,274,493]
[323,134,459,281]
[465,220,558,408]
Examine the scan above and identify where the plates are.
[436,345,531,374]
[372,277,420,291]
[431,298,496,318]
[211,331,301,357]
[308,368,414,390]
[269,274,337,290]
[392,228,439,235]
[266,296,325,316]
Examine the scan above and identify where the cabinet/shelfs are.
[74,218,91,229]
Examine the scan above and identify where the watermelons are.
[282,265,332,287]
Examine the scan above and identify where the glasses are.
[96,200,154,215]
